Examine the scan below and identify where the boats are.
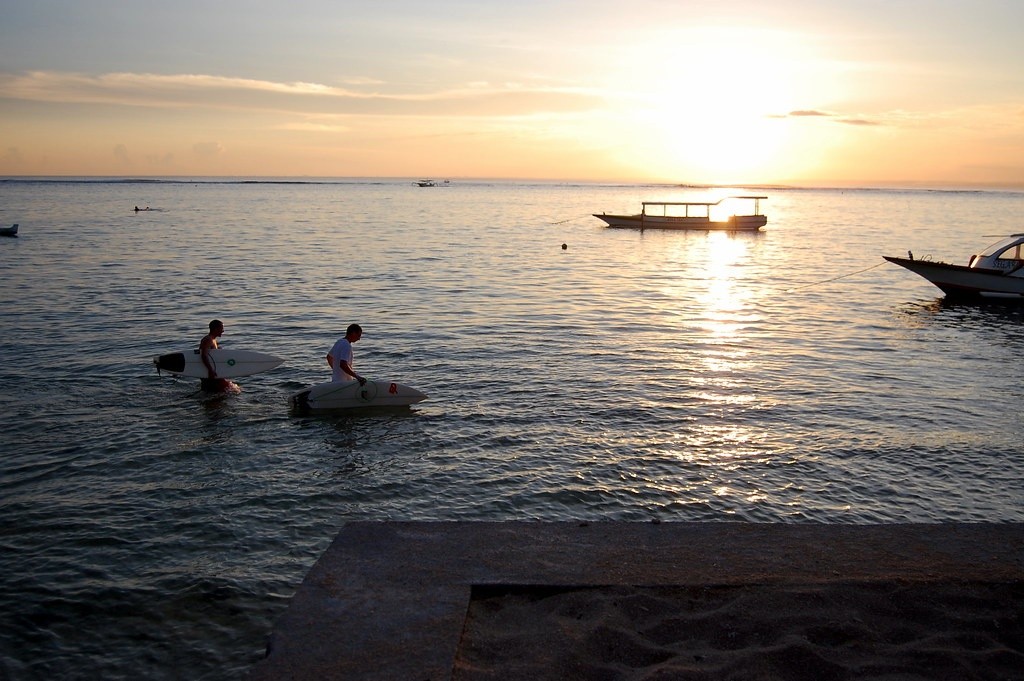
[882,234,1024,300]
[592,196,769,231]
[415,181,437,186]
[0,223,19,235]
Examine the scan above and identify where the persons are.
[326,323,367,383]
[200,320,232,385]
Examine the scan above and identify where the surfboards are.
[287,379,429,410]
[153,350,286,380]
